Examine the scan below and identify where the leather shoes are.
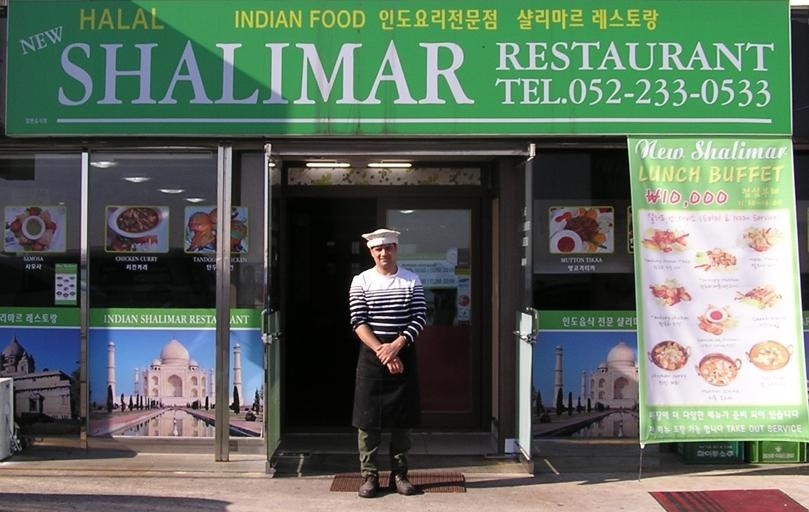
[358,481,379,497]
[389,474,416,495]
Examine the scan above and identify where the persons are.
[349,228,427,497]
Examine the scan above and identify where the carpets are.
[650,489,809,512]
[330,468,467,496]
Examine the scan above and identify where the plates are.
[550,207,612,254]
[108,206,164,239]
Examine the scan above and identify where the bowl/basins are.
[706,307,727,324]
[21,215,46,241]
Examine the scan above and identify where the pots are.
[647,340,794,387]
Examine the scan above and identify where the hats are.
[362,228,401,248]
